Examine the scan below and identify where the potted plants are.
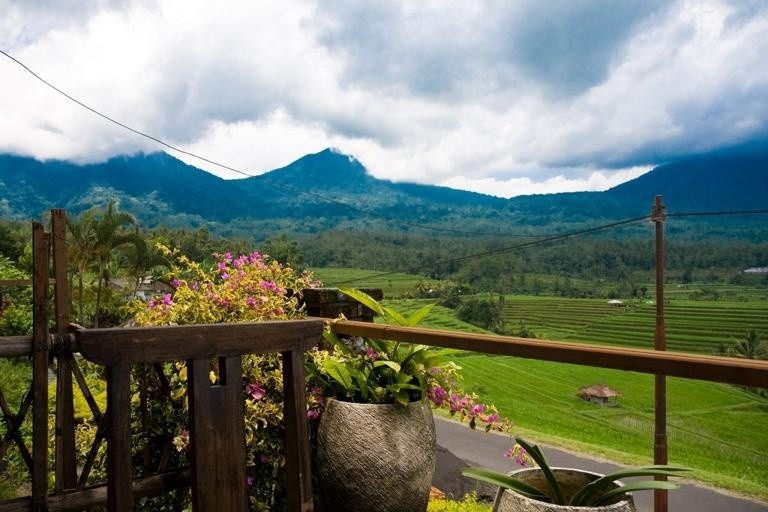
[314,286,438,512]
[461,434,692,512]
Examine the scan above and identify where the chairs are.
[75,318,328,511]
